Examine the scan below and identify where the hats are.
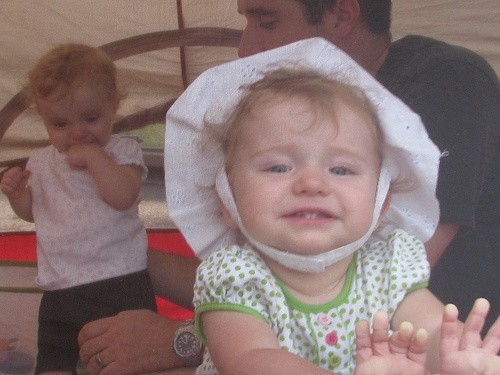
[164,35,441,263]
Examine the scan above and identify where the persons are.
[0,42,159,375]
[76,0,500,375]
[163,35,500,375]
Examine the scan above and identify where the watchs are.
[169,317,205,367]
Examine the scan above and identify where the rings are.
[95,352,106,367]
